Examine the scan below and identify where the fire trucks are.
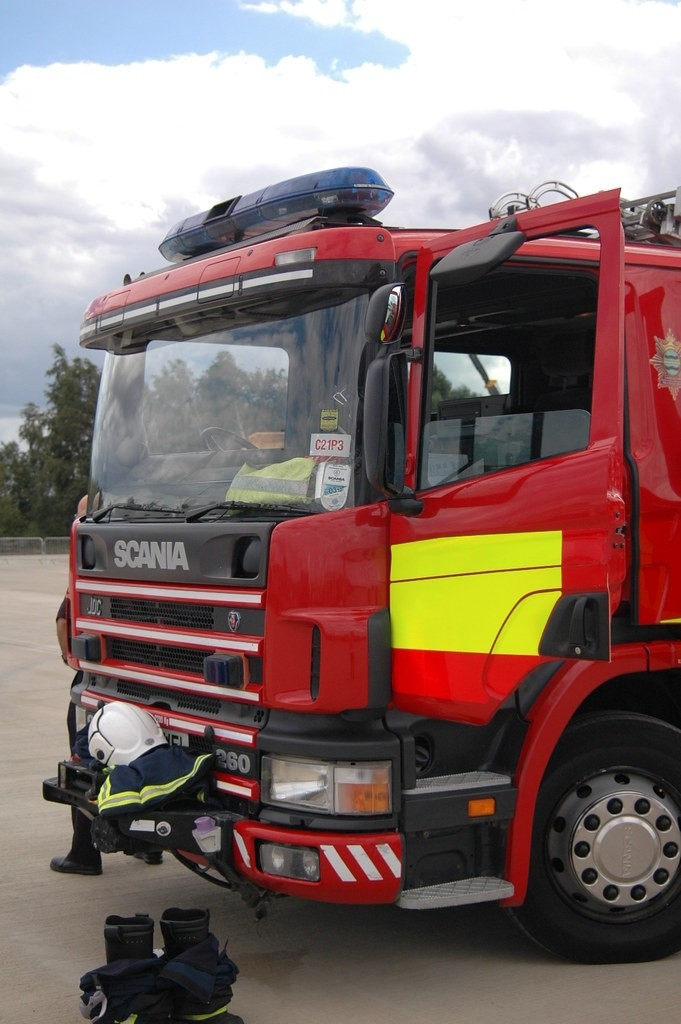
[44,167,680,964]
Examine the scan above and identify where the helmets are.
[89,701,169,767]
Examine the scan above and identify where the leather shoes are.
[124,848,164,866]
[48,852,103,875]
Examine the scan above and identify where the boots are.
[104,907,245,1024]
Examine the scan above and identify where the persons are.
[51,488,163,876]
